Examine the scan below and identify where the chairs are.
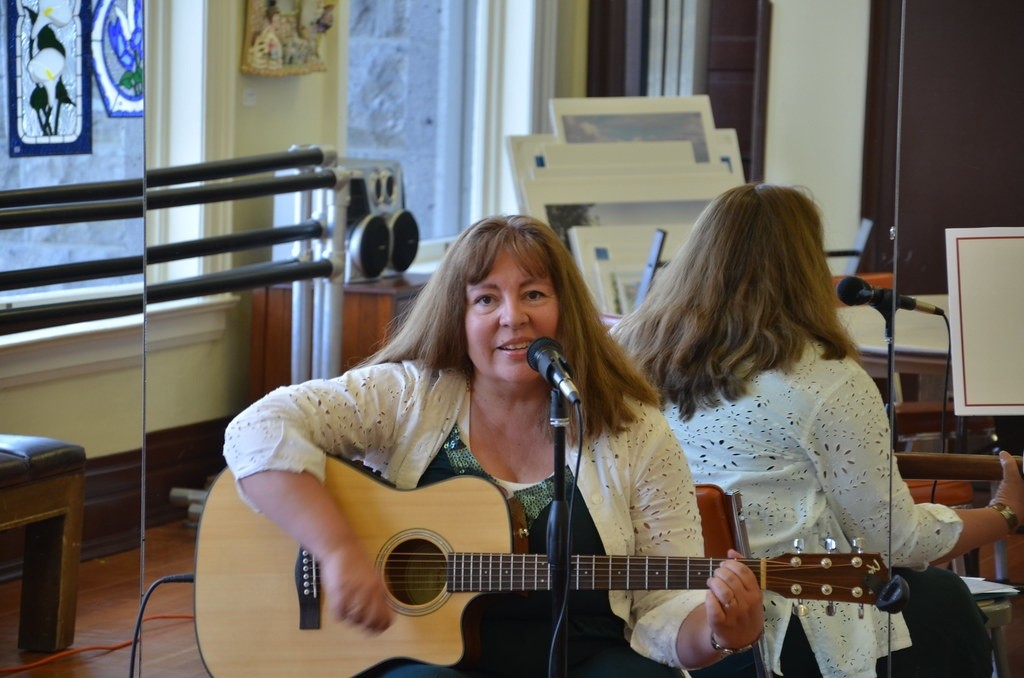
[692,483,754,560]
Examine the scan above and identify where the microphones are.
[836,276,945,316]
[525,336,581,405]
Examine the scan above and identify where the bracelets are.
[711,632,761,655]
[987,503,1019,529]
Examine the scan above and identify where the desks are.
[253,271,427,399]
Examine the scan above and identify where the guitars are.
[194,449,893,678]
[887,452,1024,483]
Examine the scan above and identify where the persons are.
[223,214,764,678]
[604,184,1024,678]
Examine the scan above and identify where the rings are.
[347,604,367,616]
[725,599,737,608]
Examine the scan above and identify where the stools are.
[0,435,85,651]
